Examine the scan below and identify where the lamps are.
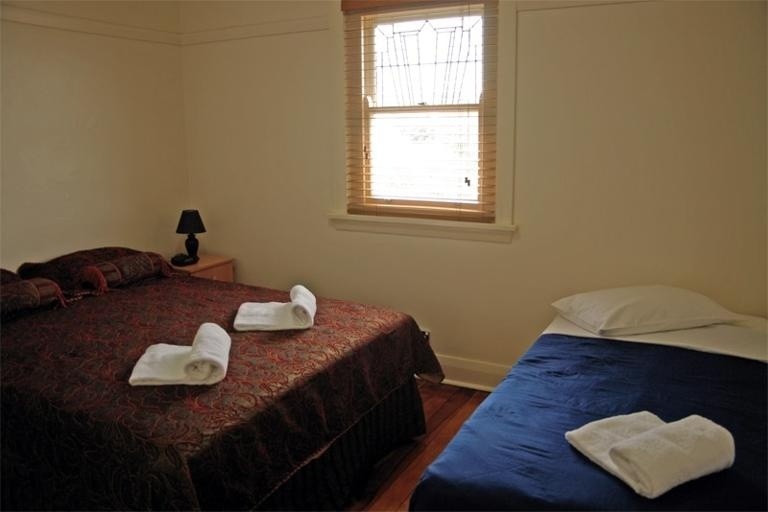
[175,208,207,258]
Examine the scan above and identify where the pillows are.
[1,252,169,322]
[550,282,748,340]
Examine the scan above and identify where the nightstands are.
[172,254,236,287]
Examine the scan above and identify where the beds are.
[412,306,768,511]
[0,250,424,512]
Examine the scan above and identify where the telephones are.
[171,253,198,266]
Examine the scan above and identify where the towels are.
[125,284,320,392]
[560,411,739,497]
